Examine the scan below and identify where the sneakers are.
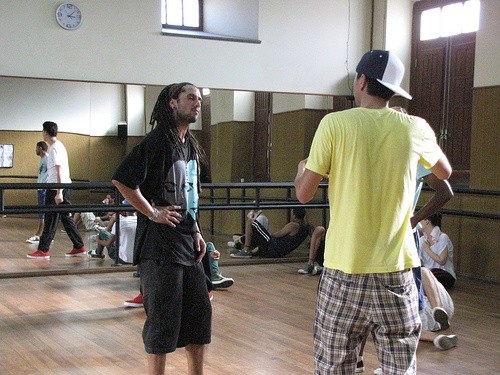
[298,263,313,274]
[26,249,51,258]
[65,244,86,257]
[208,291,214,301]
[210,272,234,288]
[124,293,144,307]
[25,234,41,243]
[88,235,99,242]
[87,250,105,259]
[311,262,322,275]
[93,225,107,232]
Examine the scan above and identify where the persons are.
[227,202,317,259]
[124,241,234,307]
[408,264,459,349]
[296,224,329,276]
[25,121,138,265]
[112,82,216,374]
[391,106,457,290]
[295,49,453,375]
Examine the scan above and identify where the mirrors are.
[1,74,410,279]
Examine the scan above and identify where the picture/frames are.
[0,144,14,168]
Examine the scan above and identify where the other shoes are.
[227,241,235,247]
[373,367,382,375]
[239,236,245,245]
[230,249,253,258]
[433,334,458,350]
[432,307,450,330]
[355,356,365,372]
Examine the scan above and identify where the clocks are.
[55,3,84,31]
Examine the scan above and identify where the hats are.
[356,50,412,100]
